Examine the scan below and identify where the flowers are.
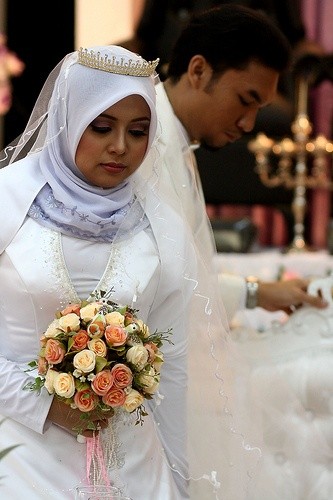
[23,285,176,438]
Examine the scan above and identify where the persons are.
[1,0,333,499]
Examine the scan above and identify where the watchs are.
[246,275,260,308]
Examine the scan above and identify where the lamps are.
[246,77,333,253]
